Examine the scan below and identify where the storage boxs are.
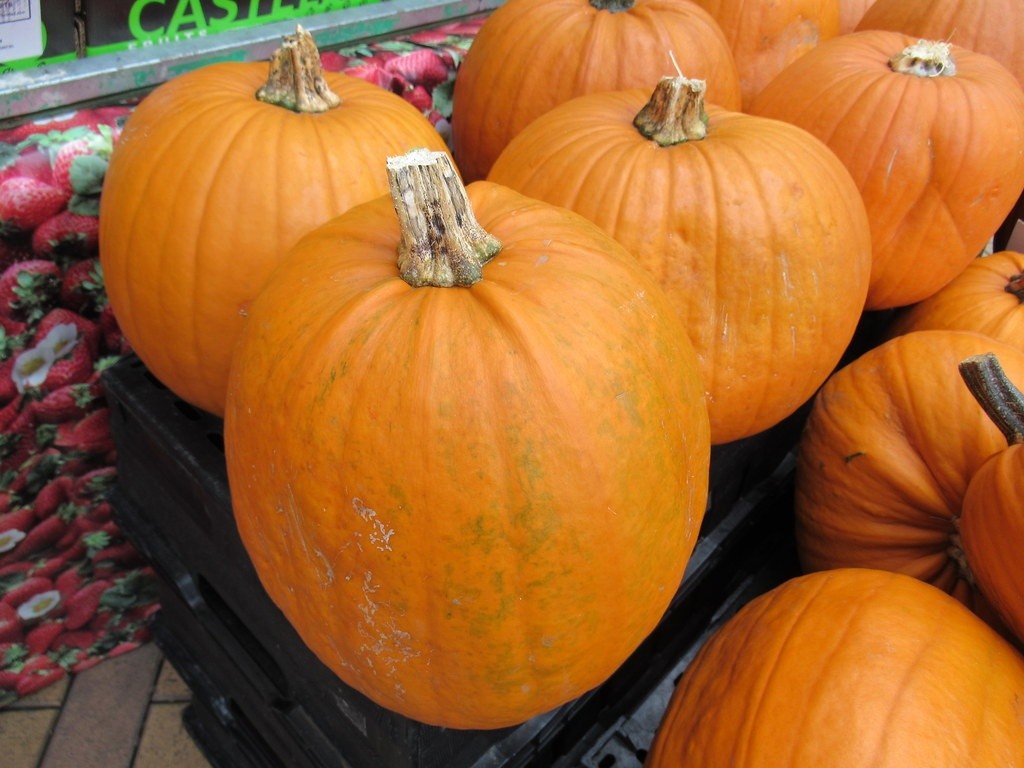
[100,302,913,768]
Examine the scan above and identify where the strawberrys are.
[0,13,484,707]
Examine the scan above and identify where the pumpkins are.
[99,0,1024,768]
